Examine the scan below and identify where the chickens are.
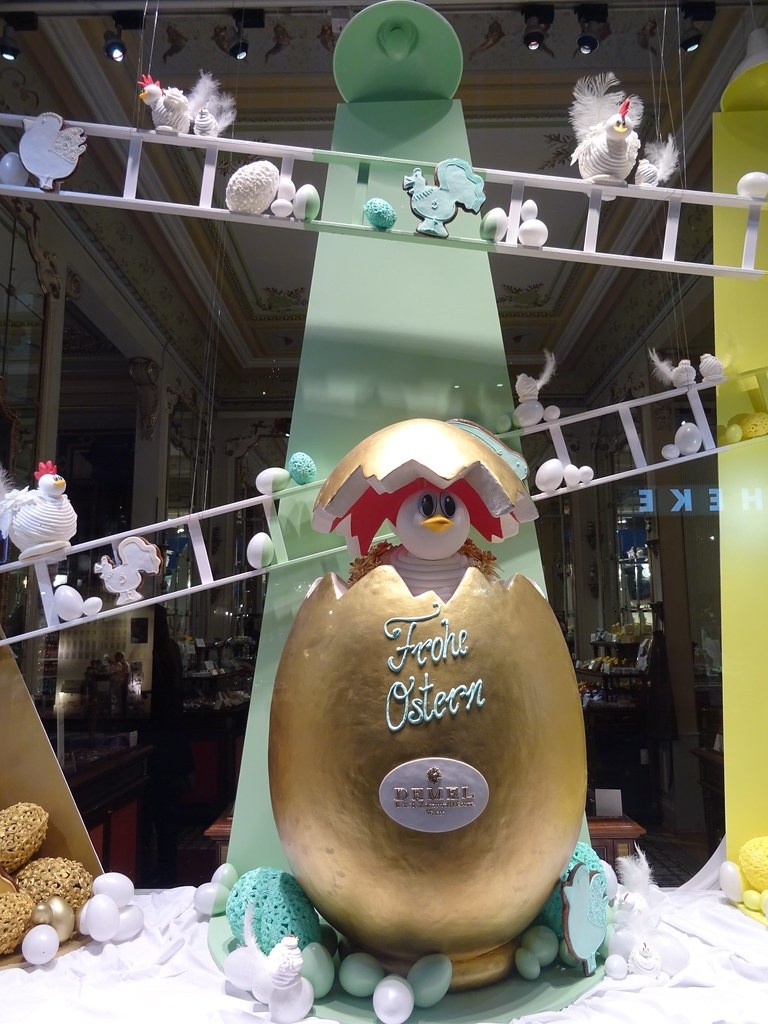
[614,888,660,979]
[516,350,556,402]
[649,345,732,387]
[0,460,78,560]
[403,158,486,238]
[567,71,681,189]
[18,112,87,189]
[136,68,237,139]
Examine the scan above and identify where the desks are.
[205,800,648,885]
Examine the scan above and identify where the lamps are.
[219,8,265,61]
[521,5,555,51]
[103,10,145,63]
[680,0,717,53]
[0,11,38,61]
[573,3,613,56]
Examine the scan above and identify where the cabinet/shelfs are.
[65,744,155,889]
[154,638,257,854]
[690,746,725,859]
[574,642,651,807]
